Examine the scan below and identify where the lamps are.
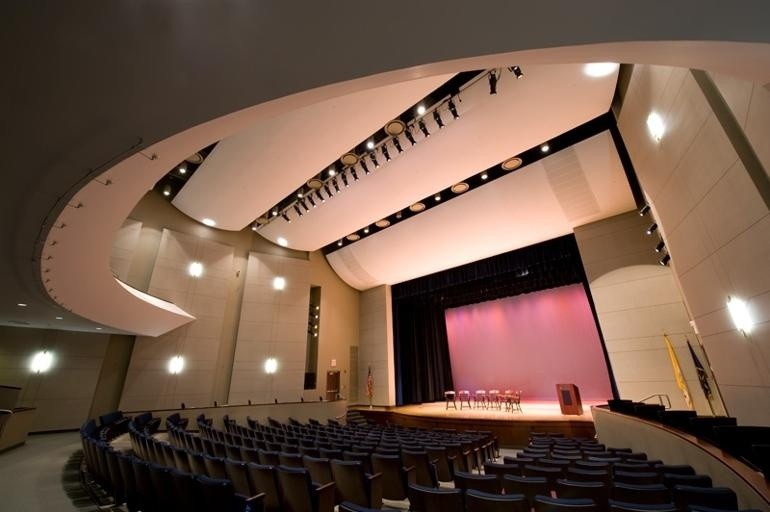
[639,203,671,268]
[308,303,320,339]
[280,66,526,225]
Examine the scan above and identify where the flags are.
[365,371,374,397]
[664,336,690,407]
[687,341,712,399]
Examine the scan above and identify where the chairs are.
[444,389,524,415]
[78,409,593,511]
[593,398,769,510]
